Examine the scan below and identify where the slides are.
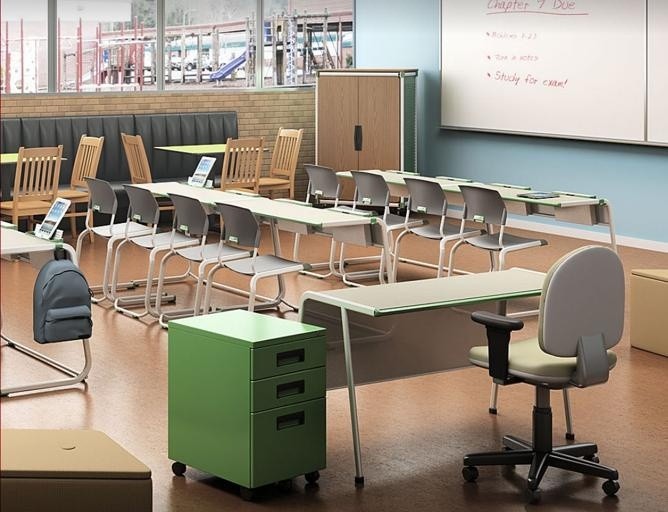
[211,50,251,81]
[76,64,108,82]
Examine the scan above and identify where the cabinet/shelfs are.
[315,71,417,206]
[168,320,327,501]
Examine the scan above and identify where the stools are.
[0,428,154,512]
[629,269,668,358]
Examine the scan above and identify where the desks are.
[298,266,575,488]
[0,220,91,398]
[334,165,620,256]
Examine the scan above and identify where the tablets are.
[35,197,71,240]
[190,157,216,187]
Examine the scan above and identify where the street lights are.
[181,8,201,84]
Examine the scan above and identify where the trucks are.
[205,49,245,72]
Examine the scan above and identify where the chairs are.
[462,244,626,505]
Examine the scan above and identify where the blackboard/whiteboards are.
[438,0,668,147]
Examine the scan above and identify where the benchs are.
[0,110,243,233]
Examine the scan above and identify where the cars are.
[170,55,207,71]
